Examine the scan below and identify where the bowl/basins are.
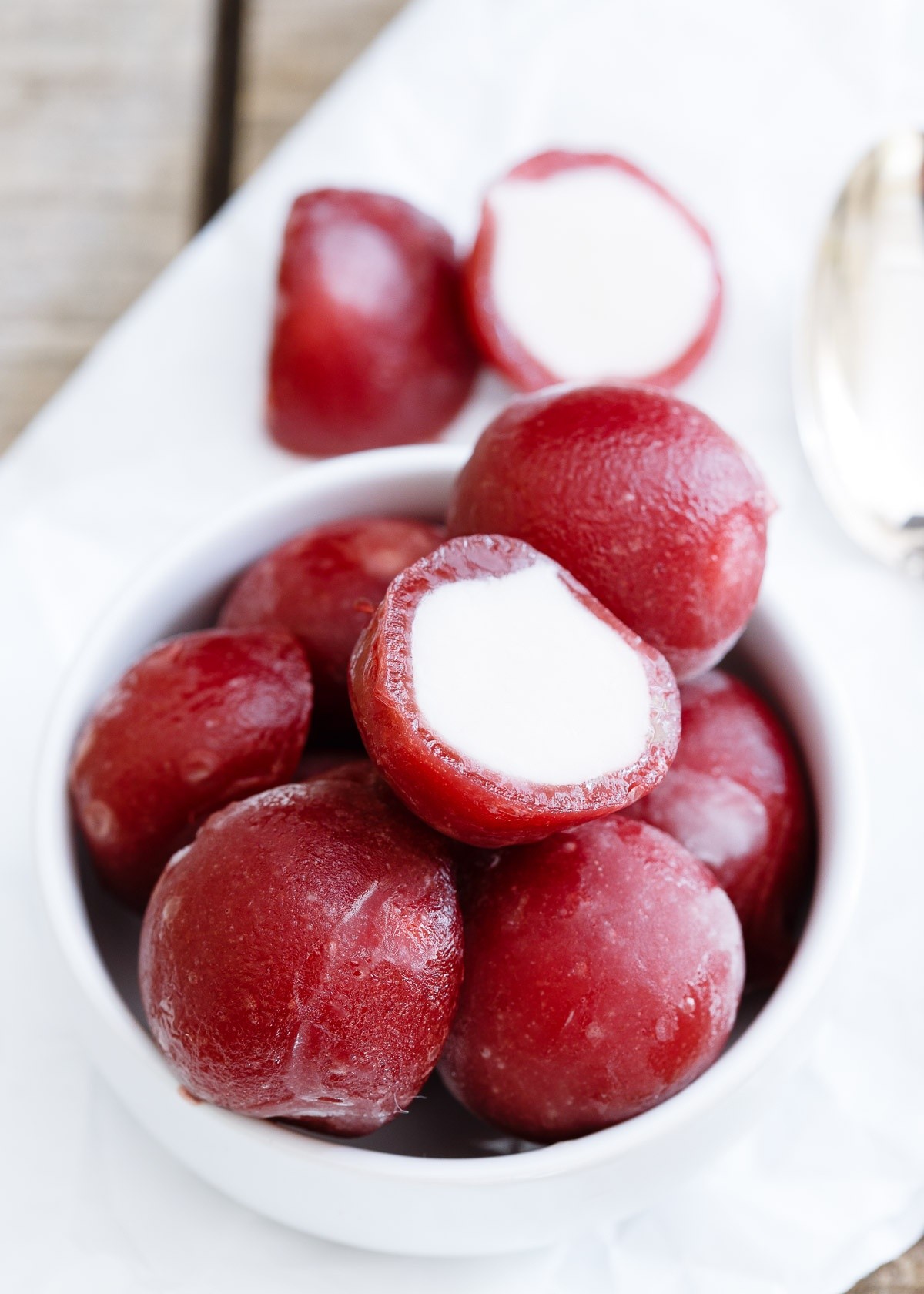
[26,443,863,1263]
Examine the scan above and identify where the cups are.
[790,90,923,565]
